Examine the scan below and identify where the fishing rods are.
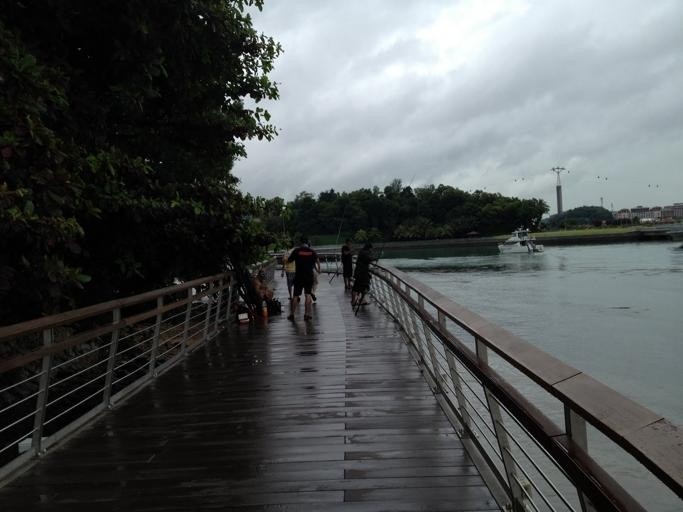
[280,217,288,277]
[353,243,385,315]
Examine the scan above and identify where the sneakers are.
[287,314,295,321]
[304,315,313,321]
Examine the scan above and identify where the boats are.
[496,224,544,253]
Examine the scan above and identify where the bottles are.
[260,300,268,317]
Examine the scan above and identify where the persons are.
[249,271,273,315]
[278,241,300,302]
[308,240,319,302]
[351,243,376,306]
[286,239,321,323]
[341,239,352,289]
